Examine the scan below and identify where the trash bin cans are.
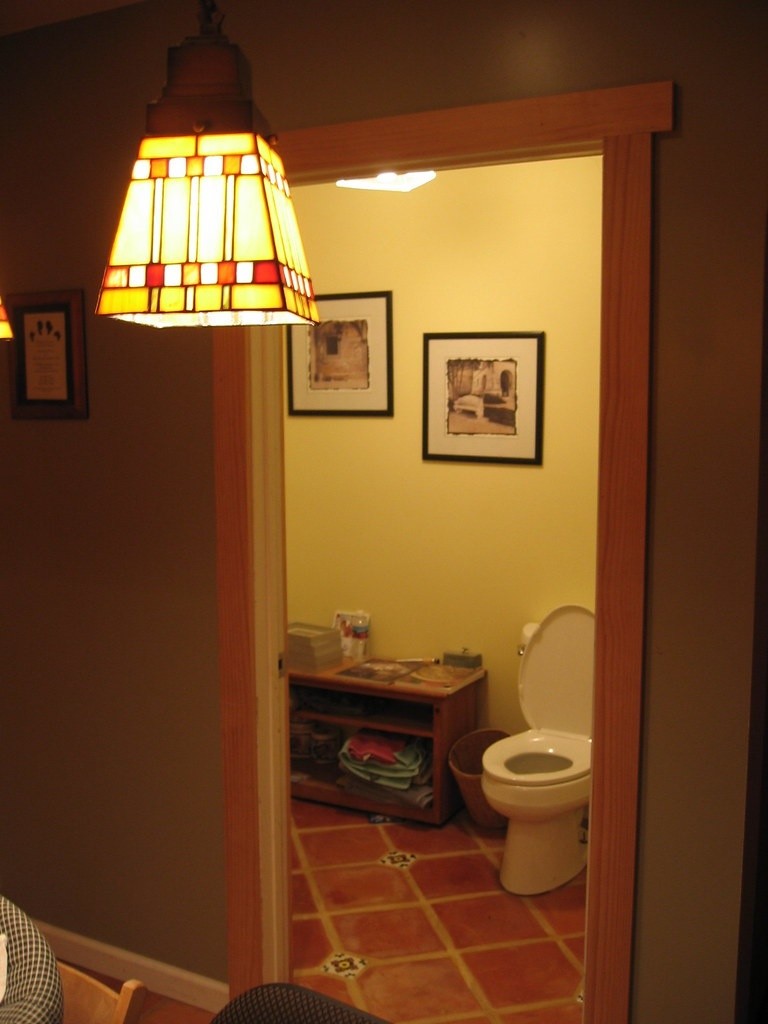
[447,728,512,828]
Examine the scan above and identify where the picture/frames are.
[6,289,90,421]
[423,331,545,464]
[286,290,394,417]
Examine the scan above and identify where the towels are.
[337,726,434,812]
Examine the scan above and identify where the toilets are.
[480,604,596,895]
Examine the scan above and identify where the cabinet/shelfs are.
[287,660,486,828]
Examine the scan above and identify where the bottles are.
[350,620,369,663]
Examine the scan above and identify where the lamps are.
[0,298,13,340]
[92,2,319,328]
